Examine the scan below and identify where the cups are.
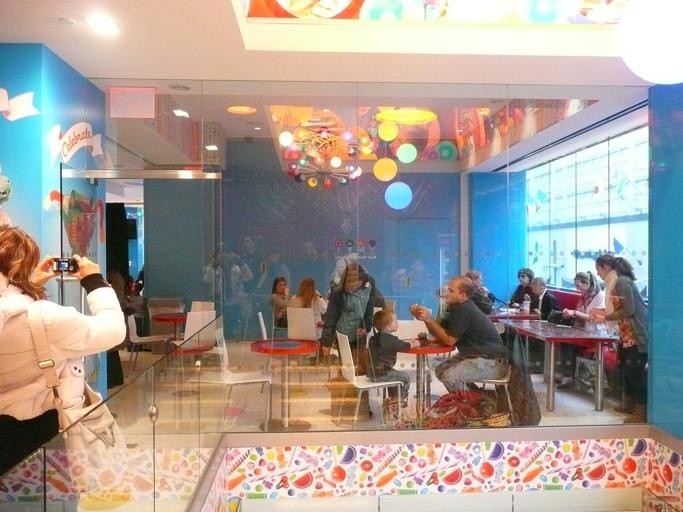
[490,308,496,317]
[522,300,531,314]
[418,333,427,346]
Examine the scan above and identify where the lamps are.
[371,105,418,211]
[283,131,360,190]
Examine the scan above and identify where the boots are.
[615,394,633,412]
[624,402,642,423]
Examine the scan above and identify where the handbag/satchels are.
[425,392,511,427]
[54,381,132,494]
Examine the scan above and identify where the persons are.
[1,226,128,512]
[410,270,510,393]
[509,255,648,426]
[270,253,418,408]
[135,264,144,352]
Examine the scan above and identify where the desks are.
[395,336,457,423]
[496,317,619,412]
[480,304,540,320]
[249,337,320,430]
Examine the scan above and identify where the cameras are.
[52,258,76,272]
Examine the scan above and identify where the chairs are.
[331,331,405,430]
[459,347,518,429]
[214,327,273,427]
[126,294,219,372]
[392,320,429,373]
[284,306,317,340]
[256,310,268,339]
